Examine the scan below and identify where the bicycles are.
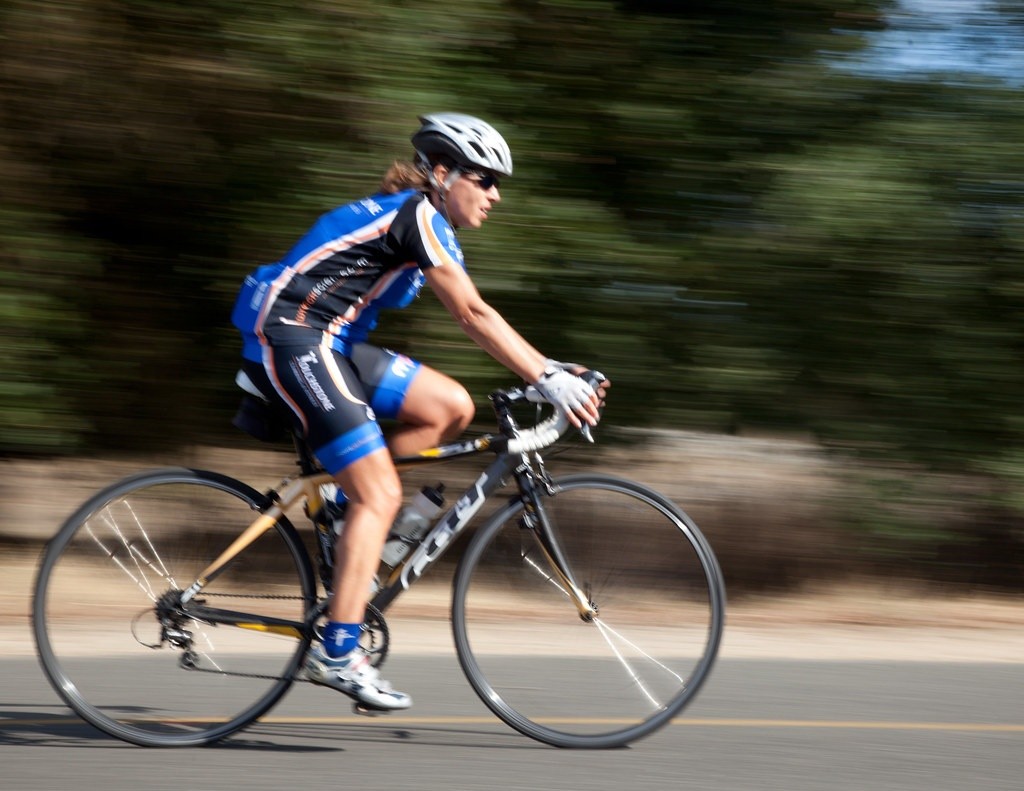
[25,367,732,752]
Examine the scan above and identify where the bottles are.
[380,482,445,567]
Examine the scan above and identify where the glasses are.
[442,160,500,189]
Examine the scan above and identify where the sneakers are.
[304,639,413,708]
[304,482,348,536]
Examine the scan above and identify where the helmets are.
[411,112,512,178]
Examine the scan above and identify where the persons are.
[234,112,610,710]
